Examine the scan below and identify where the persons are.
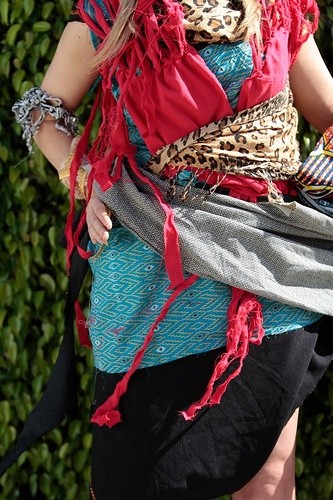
[11,1,333,500]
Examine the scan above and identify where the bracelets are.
[56,135,96,201]
